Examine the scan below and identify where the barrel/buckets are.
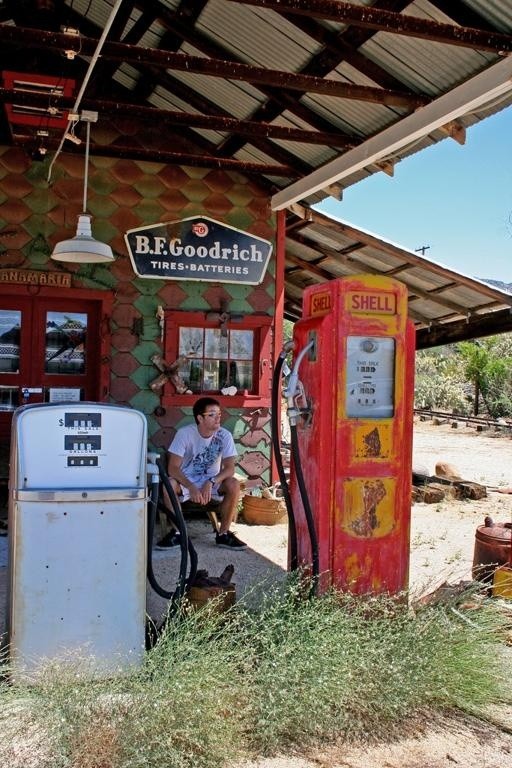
[473,522,512,592]
[184,576,237,626]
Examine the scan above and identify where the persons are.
[154,396,250,551]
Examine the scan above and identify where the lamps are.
[49,109,114,265]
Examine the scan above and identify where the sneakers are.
[215,531,247,550]
[156,527,180,549]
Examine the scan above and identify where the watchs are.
[209,476,216,486]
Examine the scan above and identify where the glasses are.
[202,412,221,417]
